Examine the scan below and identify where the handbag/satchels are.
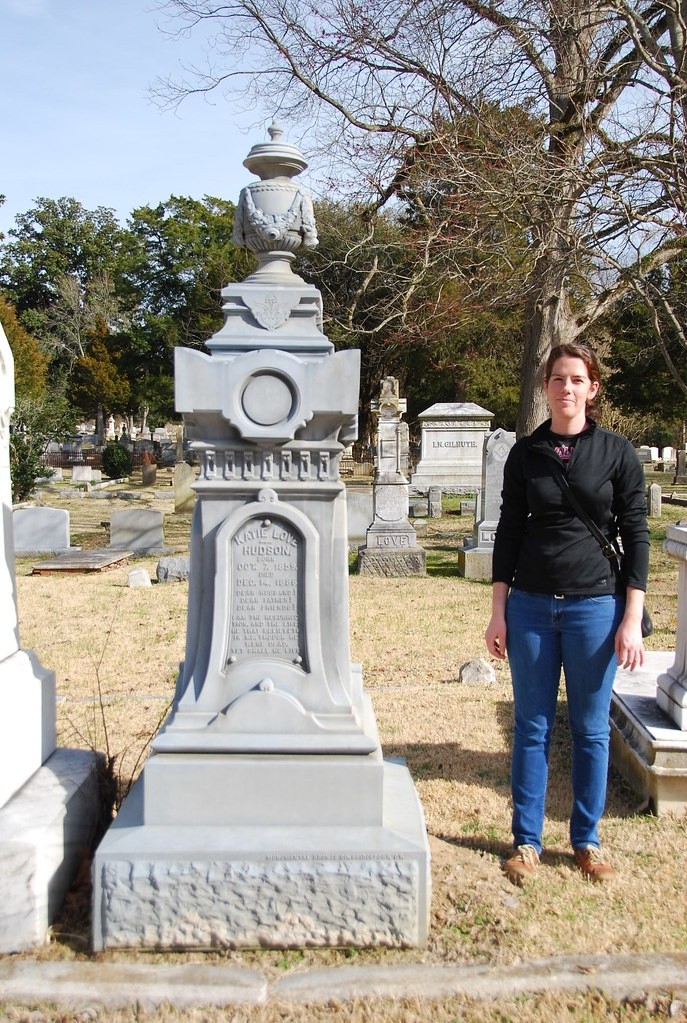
[613,551,653,638]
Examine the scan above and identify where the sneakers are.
[575,843,614,881]
[505,842,541,878]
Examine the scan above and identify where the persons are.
[484,341,650,882]
